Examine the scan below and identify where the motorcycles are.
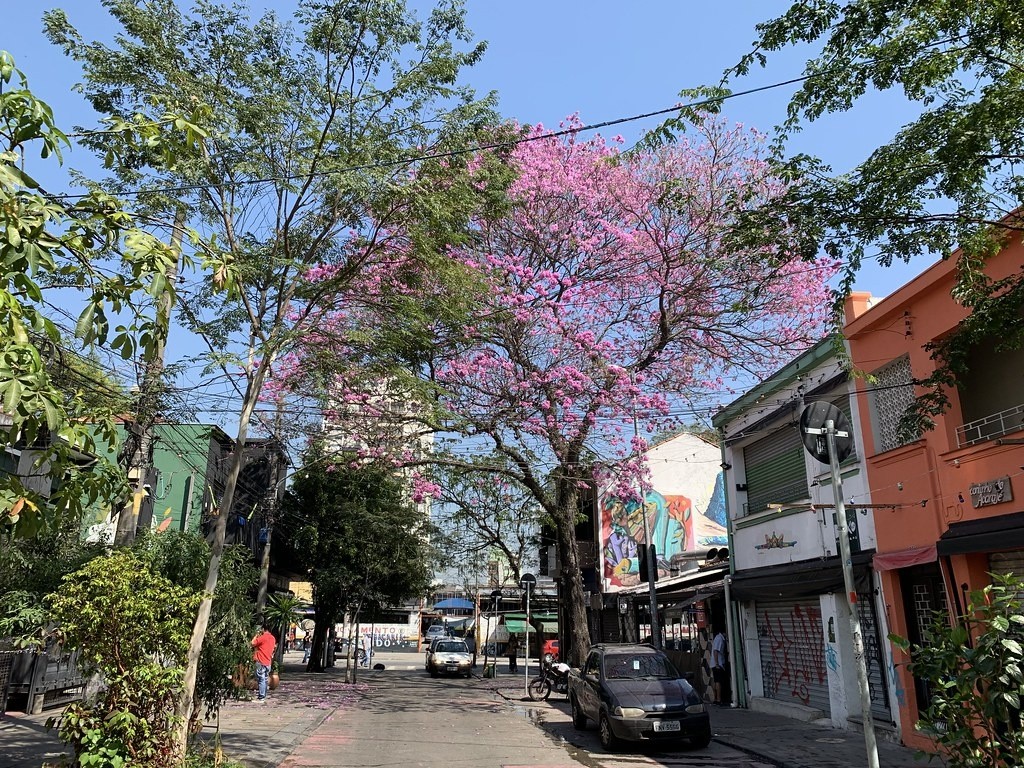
[528,653,582,701]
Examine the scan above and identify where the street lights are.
[619,336,662,650]
[253,393,361,651]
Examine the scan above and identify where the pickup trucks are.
[425,625,448,642]
[568,644,712,753]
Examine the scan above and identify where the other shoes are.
[516,669,518,672]
[712,700,724,707]
[512,670,514,674]
[251,699,264,704]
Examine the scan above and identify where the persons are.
[285,630,295,654]
[709,632,729,707]
[251,625,276,703]
[508,635,519,672]
[447,626,455,636]
[302,632,311,663]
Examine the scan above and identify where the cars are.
[334,637,375,659]
[541,639,559,663]
[425,635,472,679]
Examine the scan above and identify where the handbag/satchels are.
[506,647,515,656]
